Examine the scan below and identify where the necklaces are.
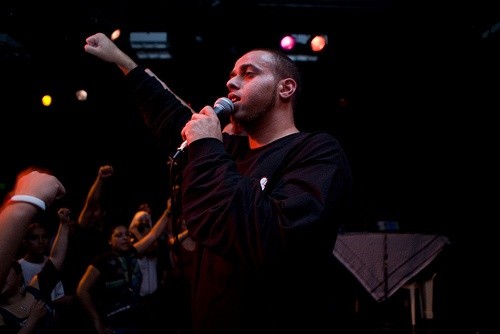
[9,301,28,315]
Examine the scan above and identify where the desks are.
[326,232,449,327]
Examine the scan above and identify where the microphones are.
[172,97,234,161]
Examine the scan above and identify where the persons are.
[84,33,350,334]
[0,165,196,334]
[77,198,172,334]
[0,208,71,334]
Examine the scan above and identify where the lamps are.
[277,31,329,55]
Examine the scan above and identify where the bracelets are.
[7,195,46,211]
[24,319,35,330]
[164,209,171,218]
